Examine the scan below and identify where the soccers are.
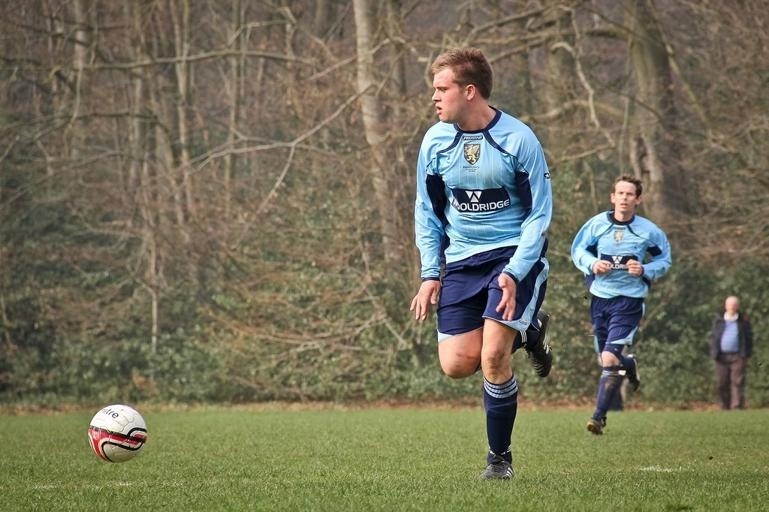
[89,403,148,464]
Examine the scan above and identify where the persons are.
[570,172,674,432]
[709,293,757,410]
[411,44,552,483]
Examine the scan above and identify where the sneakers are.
[524,311,554,378]
[625,354,641,392]
[480,459,516,481]
[588,418,605,435]
